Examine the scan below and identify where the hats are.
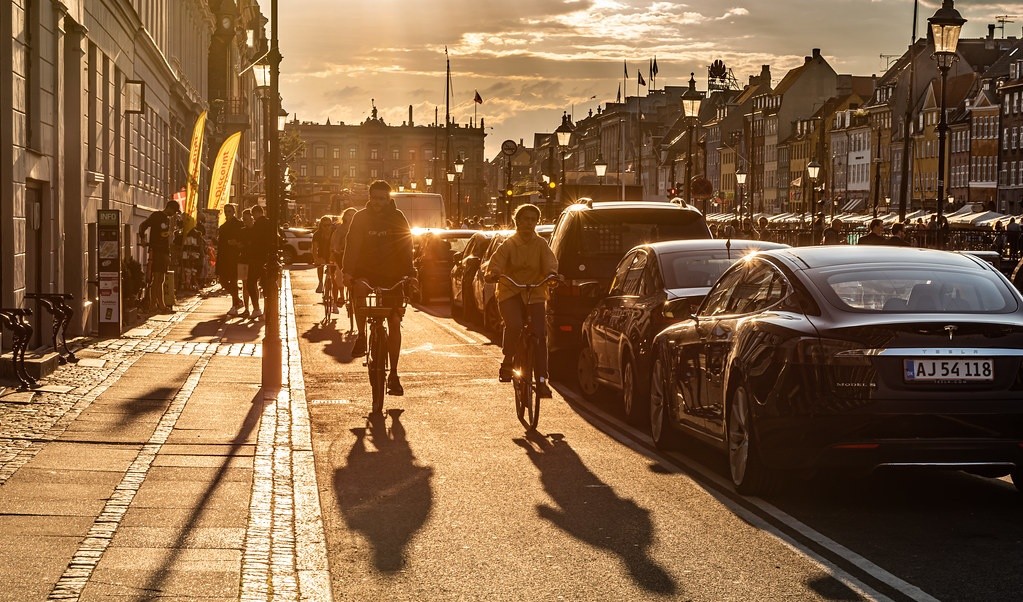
[166,201,181,214]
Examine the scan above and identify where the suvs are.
[546,197,713,385]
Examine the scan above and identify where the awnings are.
[841,198,865,212]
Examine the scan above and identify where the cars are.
[647,245,1023,495]
[578,238,796,426]
[412,228,480,306]
[279,226,314,265]
[449,227,554,333]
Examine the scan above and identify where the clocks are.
[222,16,232,29]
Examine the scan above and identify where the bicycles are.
[314,262,340,319]
[345,279,414,415]
[486,274,568,429]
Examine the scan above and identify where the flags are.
[209,132,242,228]
[185,110,206,234]
[624,60,628,79]
[649,60,658,81]
[474,91,482,104]
[637,69,646,86]
[617,85,620,101]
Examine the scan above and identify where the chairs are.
[883,298,907,310]
[909,284,944,311]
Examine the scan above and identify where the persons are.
[139,201,181,314]
[483,204,559,399]
[813,213,950,309]
[709,217,768,241]
[312,207,366,314]
[215,204,271,319]
[994,218,1023,261]
[340,181,418,397]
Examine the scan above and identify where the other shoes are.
[237,312,250,317]
[227,301,244,315]
[248,309,263,319]
[388,375,404,393]
[499,357,512,382]
[337,298,344,308]
[159,306,175,315]
[351,336,367,357]
[538,384,552,397]
[316,285,323,292]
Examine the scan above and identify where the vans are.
[390,192,446,235]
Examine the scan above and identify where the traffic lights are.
[667,182,683,200]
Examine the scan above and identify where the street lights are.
[735,165,747,229]
[555,111,573,201]
[927,0,968,252]
[680,72,704,204]
[807,151,820,246]
[424,172,433,193]
[446,169,455,220]
[594,154,608,185]
[454,155,464,227]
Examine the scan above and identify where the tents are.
[706,205,1023,231]
